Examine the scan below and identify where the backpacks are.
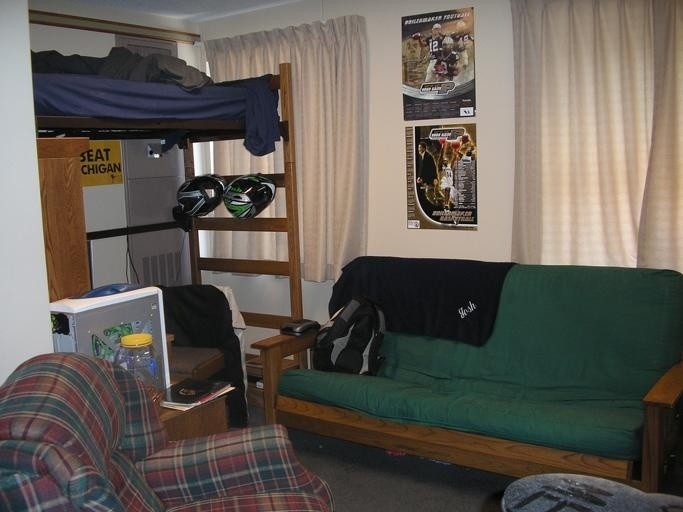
[311,297,386,377]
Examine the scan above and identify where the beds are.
[32,63,303,410]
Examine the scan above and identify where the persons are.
[416,130,475,201]
[412,20,473,82]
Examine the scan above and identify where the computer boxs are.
[49,283,171,393]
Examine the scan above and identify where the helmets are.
[442,37,454,47]
[456,21,466,32]
[176,173,226,220]
[222,173,277,221]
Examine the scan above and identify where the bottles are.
[115,334,159,413]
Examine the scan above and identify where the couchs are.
[0,352,335,512]
[251,256,683,493]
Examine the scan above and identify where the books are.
[161,376,236,412]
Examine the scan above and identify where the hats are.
[431,23,441,30]
